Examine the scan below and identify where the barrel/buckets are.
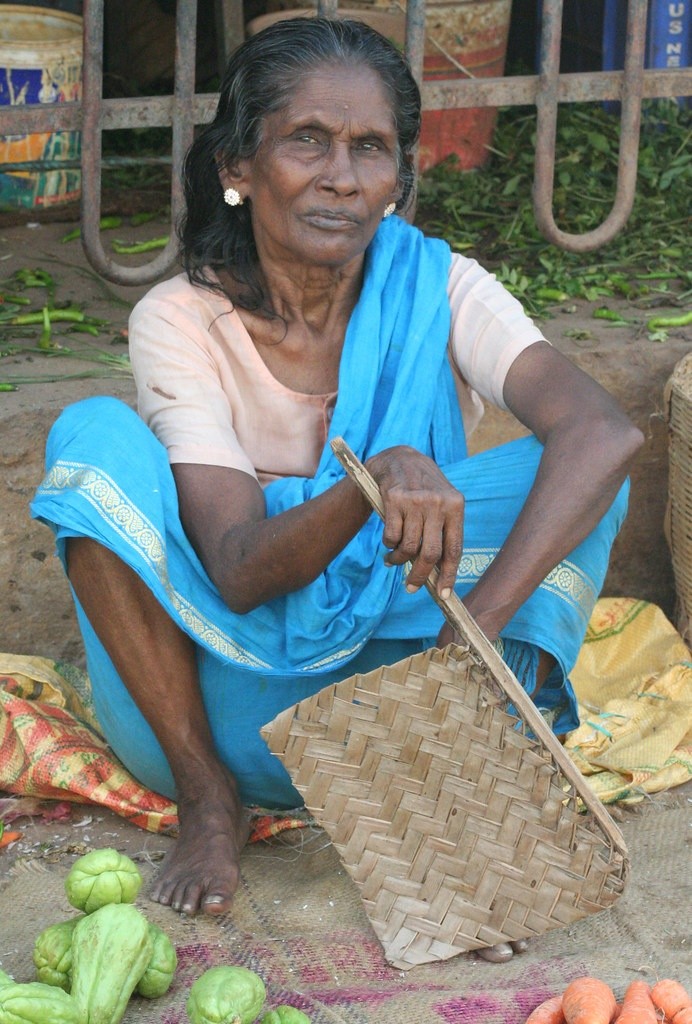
[299,0,515,172]
[2,4,84,210]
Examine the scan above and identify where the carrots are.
[525,979,692,1024]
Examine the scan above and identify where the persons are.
[31,17,641,965]
